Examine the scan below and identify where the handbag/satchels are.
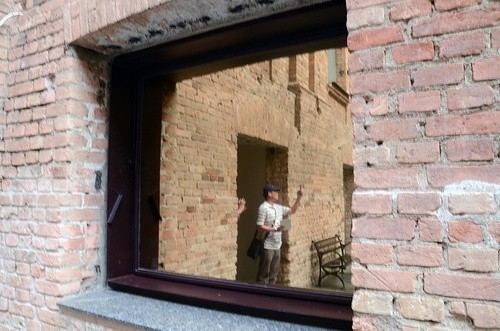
[246,238,262,260]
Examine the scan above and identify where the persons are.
[237,197,247,223]
[255,183,302,286]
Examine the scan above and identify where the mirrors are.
[105,1,354,330]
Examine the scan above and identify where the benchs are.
[309,234,352,290]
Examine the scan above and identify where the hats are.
[263,184,280,192]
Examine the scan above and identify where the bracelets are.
[274,227,278,232]
